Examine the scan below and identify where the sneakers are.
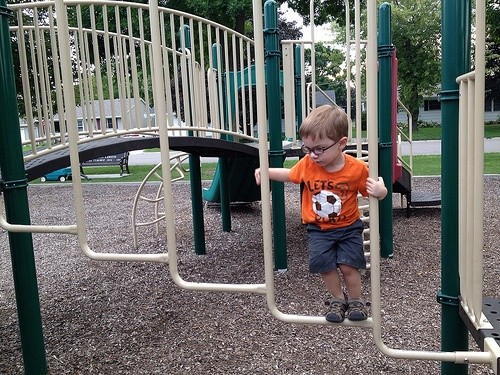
[326,292,349,322]
[347,300,368,321]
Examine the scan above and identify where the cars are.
[39,166,72,183]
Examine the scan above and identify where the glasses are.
[301,136,344,154]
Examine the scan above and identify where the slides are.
[202,131,263,203]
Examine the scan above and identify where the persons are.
[255,105,388,322]
